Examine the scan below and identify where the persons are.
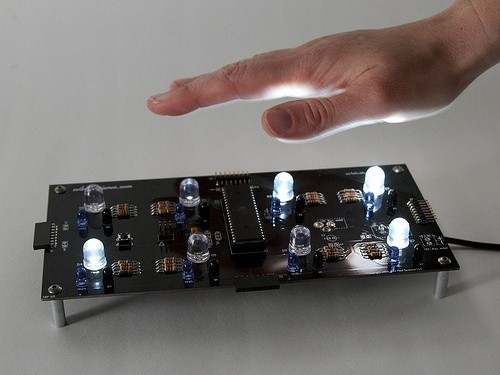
[146,0,500,144]
